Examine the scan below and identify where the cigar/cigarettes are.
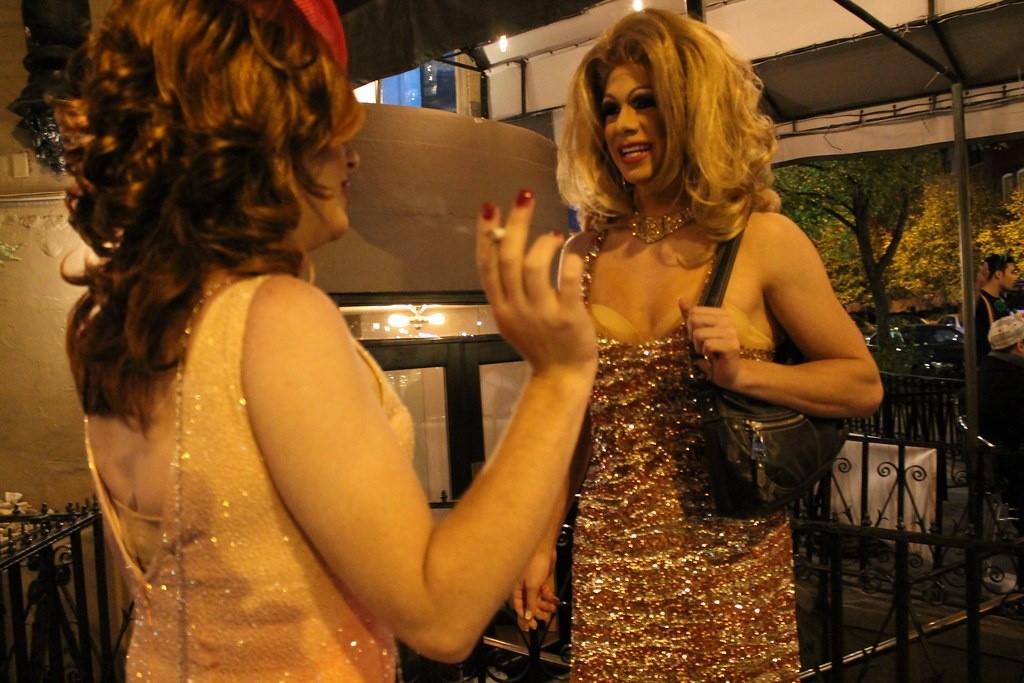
[487,228,505,242]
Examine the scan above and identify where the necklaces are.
[626,207,694,243]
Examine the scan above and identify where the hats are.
[988,316,1024,350]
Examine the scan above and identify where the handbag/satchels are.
[689,216,847,518]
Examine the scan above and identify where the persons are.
[46,0,599,683]
[960,252,1024,449]
[510,8,886,682]
